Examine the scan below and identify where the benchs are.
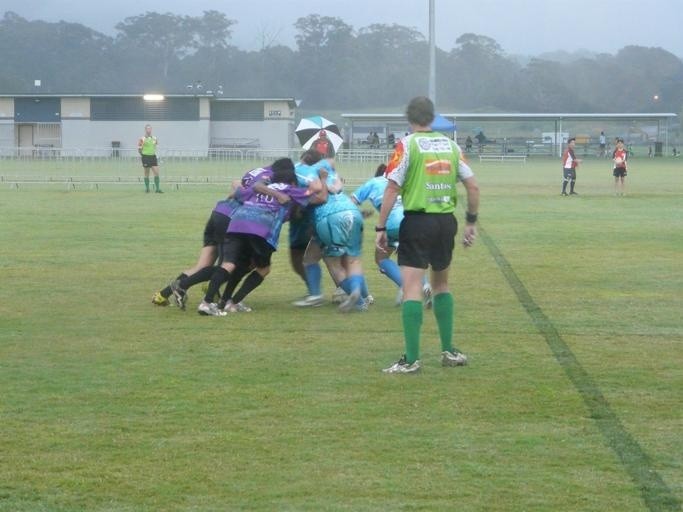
[479,155,526,162]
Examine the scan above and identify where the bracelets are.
[376,227,386,232]
[465,214,478,223]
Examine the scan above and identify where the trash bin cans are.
[654,141,663,156]
[112,141,120,157]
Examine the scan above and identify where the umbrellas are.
[296,117,344,155]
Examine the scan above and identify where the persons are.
[152,157,327,317]
[465,136,472,151]
[648,143,652,157]
[628,143,634,158]
[138,125,161,192]
[373,96,480,372]
[351,164,433,310]
[612,139,627,192]
[562,137,579,196]
[288,149,373,312]
[476,131,485,152]
[599,130,605,155]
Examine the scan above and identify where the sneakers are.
[382,355,422,373]
[291,286,374,312]
[151,279,254,316]
[441,350,467,367]
[423,284,432,310]
